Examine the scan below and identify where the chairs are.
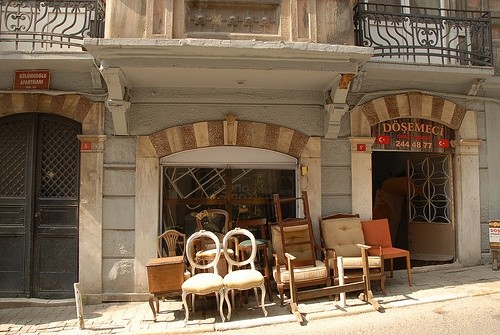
[236,218,267,241]
[222,227,269,322]
[195,209,229,278]
[272,190,316,270]
[148,229,186,322]
[268,219,334,306]
[180,229,225,327]
[319,213,388,301]
[361,219,412,287]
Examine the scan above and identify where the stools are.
[238,239,273,304]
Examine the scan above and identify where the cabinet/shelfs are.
[271,223,314,267]
[145,255,185,294]
